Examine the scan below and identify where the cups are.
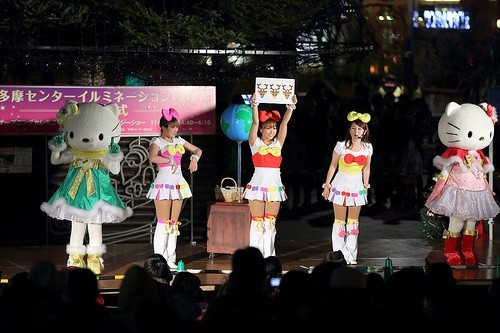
[237,187,244,203]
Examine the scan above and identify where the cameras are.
[270,276,283,286]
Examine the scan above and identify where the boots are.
[345,222,360,265]
[249,220,264,253]
[263,217,277,258]
[153,222,169,263]
[167,224,179,269]
[332,224,349,265]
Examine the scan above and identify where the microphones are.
[358,137,364,139]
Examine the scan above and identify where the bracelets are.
[190,155,198,162]
[286,105,296,110]
[322,182,330,189]
[250,101,259,107]
[169,157,174,165]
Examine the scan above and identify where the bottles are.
[177,260,184,270]
[385,257,392,273]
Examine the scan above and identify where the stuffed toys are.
[40,98,133,274]
[425,102,500,267]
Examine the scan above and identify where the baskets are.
[214,177,245,202]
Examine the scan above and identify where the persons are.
[321,111,373,264]
[0,246,500,333]
[146,108,202,268]
[243,93,297,258]
